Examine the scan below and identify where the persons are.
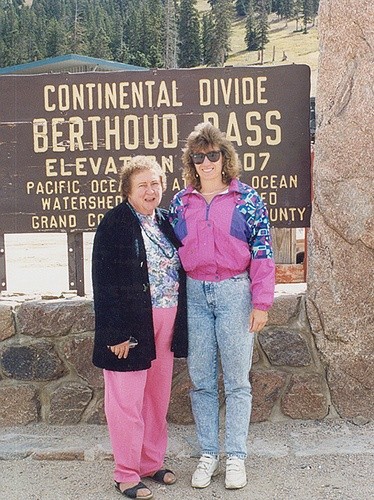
[168,121,275,489]
[93,156,189,500]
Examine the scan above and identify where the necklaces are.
[126,203,174,258]
[202,184,229,195]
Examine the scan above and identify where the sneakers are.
[225,456,247,489]
[192,454,220,487]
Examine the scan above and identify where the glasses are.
[189,150,223,164]
[129,337,139,348]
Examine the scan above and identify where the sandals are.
[146,469,177,484]
[114,481,153,499]
